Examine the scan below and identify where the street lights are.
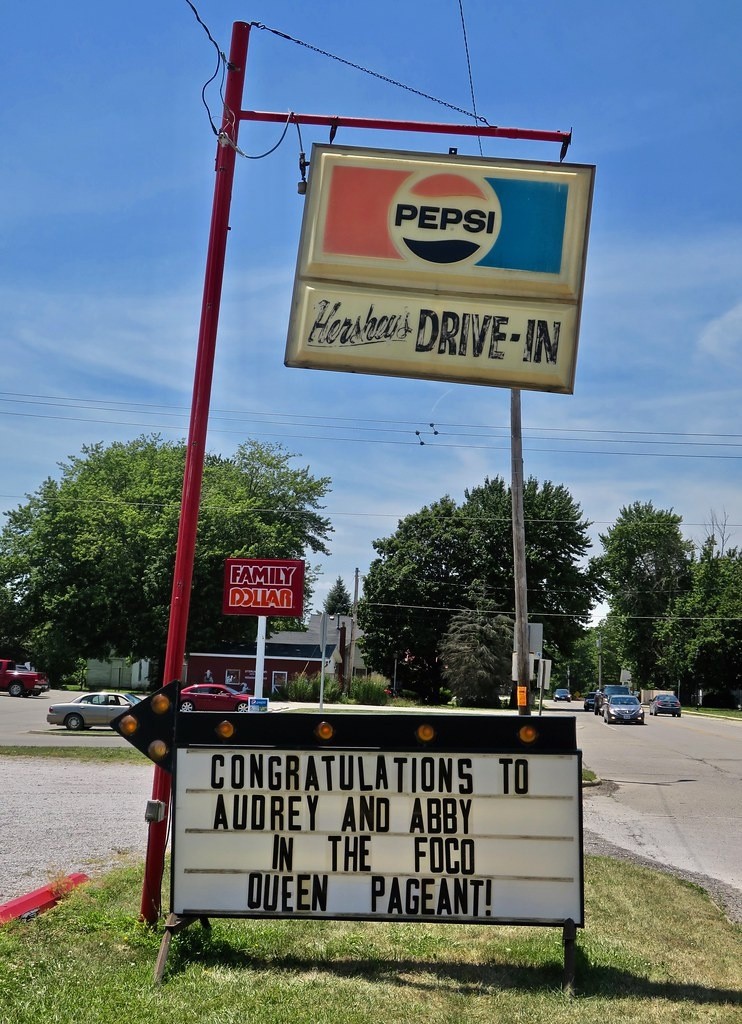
[393,651,399,691]
[330,613,346,694]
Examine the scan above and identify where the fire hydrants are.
[696,702,699,711]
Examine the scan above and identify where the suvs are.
[583,693,596,711]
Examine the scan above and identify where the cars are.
[649,695,682,718]
[47,689,142,729]
[603,695,644,725]
[553,689,572,702]
[181,683,253,712]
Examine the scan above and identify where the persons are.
[275,675,284,693]
[240,680,251,693]
[629,699,636,705]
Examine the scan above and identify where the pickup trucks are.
[0,659,50,698]
[594,685,632,716]
